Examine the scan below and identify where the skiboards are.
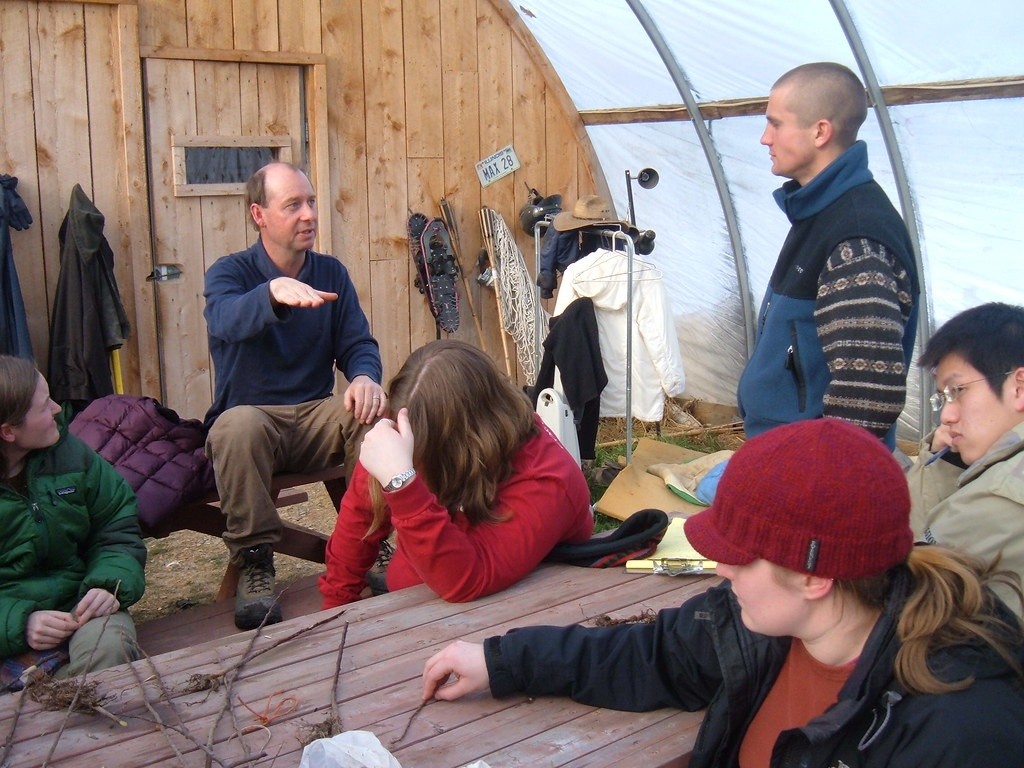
[405,211,460,333]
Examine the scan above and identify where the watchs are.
[382,468,416,493]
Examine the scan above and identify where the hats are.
[553,194,630,235]
[682,414,914,582]
[547,508,668,572]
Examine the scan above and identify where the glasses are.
[928,367,1018,414]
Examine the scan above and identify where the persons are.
[322,339,594,608]
[420,416,1024,768]
[735,60,919,474]
[0,354,148,695]
[201,160,395,630]
[903,301,1024,621]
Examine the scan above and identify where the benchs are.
[137,461,349,564]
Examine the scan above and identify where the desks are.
[0,528,724,768]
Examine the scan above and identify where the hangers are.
[572,231,663,282]
[572,230,656,285]
[573,231,657,282]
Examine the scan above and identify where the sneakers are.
[365,539,395,595]
[234,541,284,631]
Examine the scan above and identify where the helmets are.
[518,195,561,238]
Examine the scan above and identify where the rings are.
[372,397,380,399]
